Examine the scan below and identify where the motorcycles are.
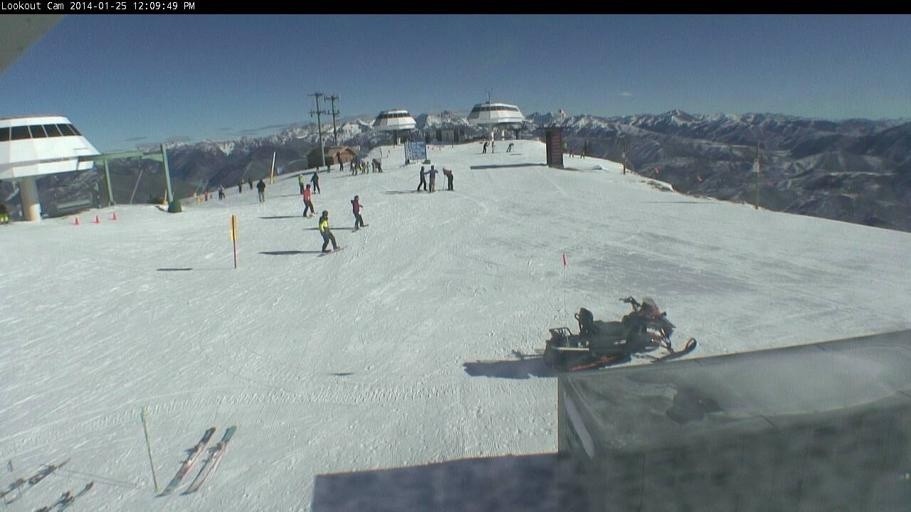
[544,297,696,374]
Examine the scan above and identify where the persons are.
[424,165,438,192]
[318,210,340,252]
[506,142,514,152]
[481,141,490,153]
[191,176,253,203]
[350,194,369,231]
[338,157,344,171]
[298,161,330,217]
[562,141,585,159]
[492,141,497,153]
[417,166,428,192]
[349,155,384,176]
[442,168,454,190]
[256,178,266,202]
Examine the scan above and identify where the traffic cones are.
[74,217,80,224]
[96,216,100,223]
[112,212,118,220]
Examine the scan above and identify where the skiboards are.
[157,425,236,496]
[316,245,347,257]
[352,224,369,233]
